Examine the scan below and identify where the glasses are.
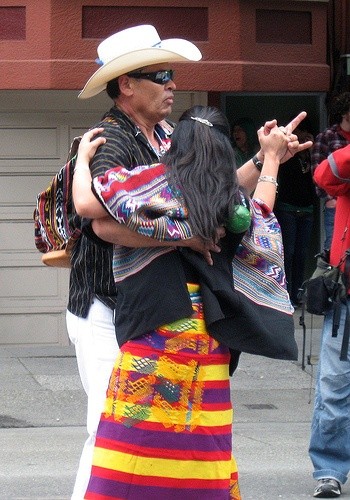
[124,69,173,84]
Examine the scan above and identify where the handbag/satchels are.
[297,253,350,316]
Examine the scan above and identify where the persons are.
[230,118,256,166]
[65,24,313,500]
[311,92,350,301]
[308,145,350,496]
[71,105,288,500]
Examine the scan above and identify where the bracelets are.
[256,175,279,186]
[73,165,91,172]
[252,156,262,169]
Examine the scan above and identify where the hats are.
[78,25,202,100]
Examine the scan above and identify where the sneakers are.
[313,478,341,497]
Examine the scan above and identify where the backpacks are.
[34,136,85,267]
